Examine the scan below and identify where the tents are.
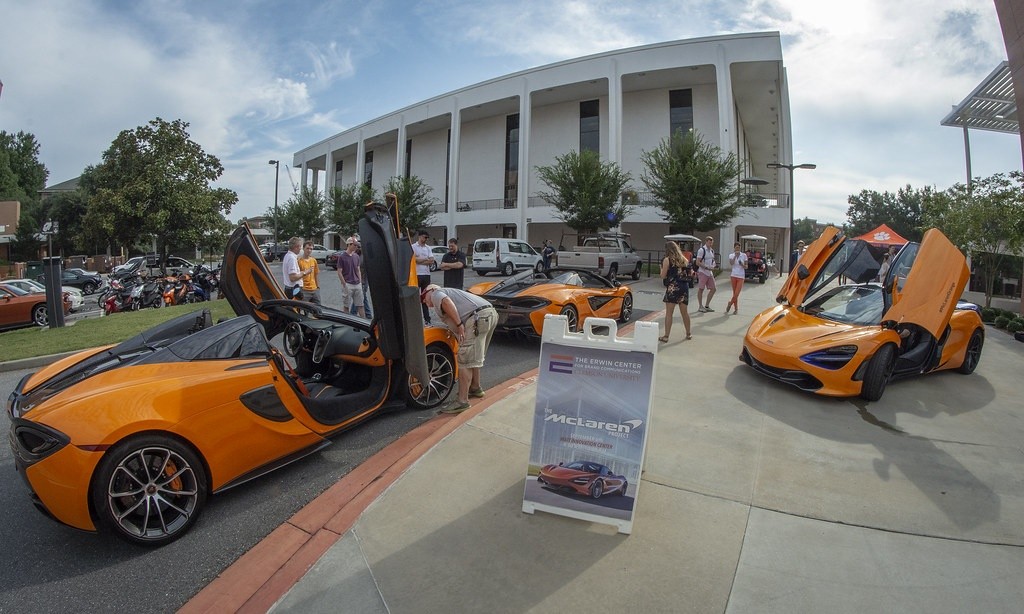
[849,223,908,248]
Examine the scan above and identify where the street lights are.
[267,159,281,259]
[951,104,991,293]
[765,161,818,274]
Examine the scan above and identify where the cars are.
[127,256,213,279]
[0,283,73,328]
[428,246,468,273]
[0,277,86,314]
[259,238,291,261]
[109,256,144,281]
[325,250,346,270]
[63,266,104,288]
[31,269,98,295]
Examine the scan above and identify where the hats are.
[346,237,361,246]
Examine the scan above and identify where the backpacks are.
[693,247,705,271]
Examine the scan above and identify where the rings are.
[461,335,462,336]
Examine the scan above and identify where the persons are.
[658,241,693,342]
[412,230,434,327]
[336,237,365,318]
[542,240,555,269]
[350,248,372,319]
[421,284,499,414]
[726,242,748,314]
[789,240,805,275]
[440,238,467,290]
[879,245,896,282]
[283,236,313,300]
[297,241,321,304]
[696,236,716,313]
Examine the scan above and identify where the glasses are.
[424,237,430,239]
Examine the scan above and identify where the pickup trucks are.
[555,230,643,285]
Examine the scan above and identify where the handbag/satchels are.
[663,257,678,286]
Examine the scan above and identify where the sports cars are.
[537,460,629,501]
[4,191,461,549]
[465,265,635,340]
[738,224,987,403]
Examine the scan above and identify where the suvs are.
[296,244,337,264]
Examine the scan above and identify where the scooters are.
[96,258,226,316]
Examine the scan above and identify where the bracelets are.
[304,272,306,275]
[457,322,462,327]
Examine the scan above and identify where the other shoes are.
[441,401,471,413]
[734,309,737,315]
[699,306,707,313]
[705,306,715,312]
[726,302,732,312]
[659,335,668,342]
[687,334,692,339]
[457,386,485,398]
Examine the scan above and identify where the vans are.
[471,236,545,277]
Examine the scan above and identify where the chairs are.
[509,245,522,253]
[588,244,597,247]
[600,244,611,247]
